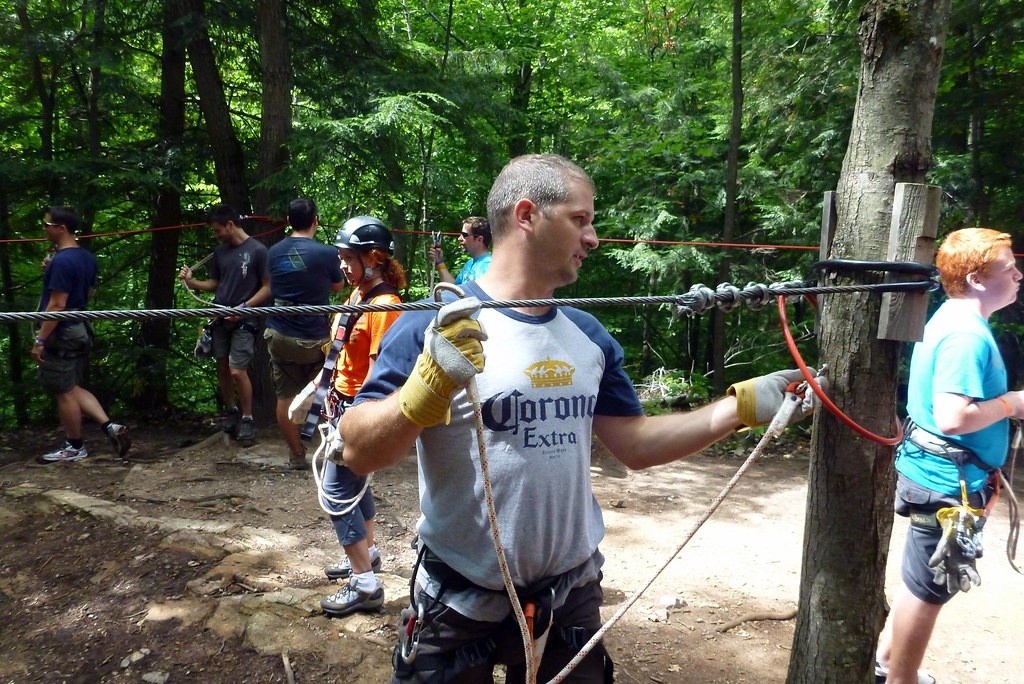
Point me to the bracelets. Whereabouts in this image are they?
[35,338,44,346]
[239,303,243,307]
[436,260,446,270]
[996,396,1011,416]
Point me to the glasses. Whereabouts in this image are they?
[461,232,486,238]
[42,218,59,228]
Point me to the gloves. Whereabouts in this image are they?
[327,415,348,466]
[726,367,830,434]
[928,507,983,594]
[399,296,488,428]
[288,381,318,424]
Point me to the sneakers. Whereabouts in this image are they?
[36,441,88,465]
[324,547,382,578]
[320,570,384,614]
[104,423,131,458]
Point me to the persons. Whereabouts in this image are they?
[338,153,828,684]
[263,198,344,470]
[428,216,492,285]
[876,228,1024,684]
[31,206,132,463]
[288,216,407,613]
[177,205,272,440]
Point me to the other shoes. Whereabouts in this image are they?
[221,406,241,432]
[287,447,306,469]
[238,417,256,439]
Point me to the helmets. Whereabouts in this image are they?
[334,216,394,257]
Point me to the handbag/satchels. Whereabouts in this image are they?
[45,308,89,350]
[194,323,213,360]
